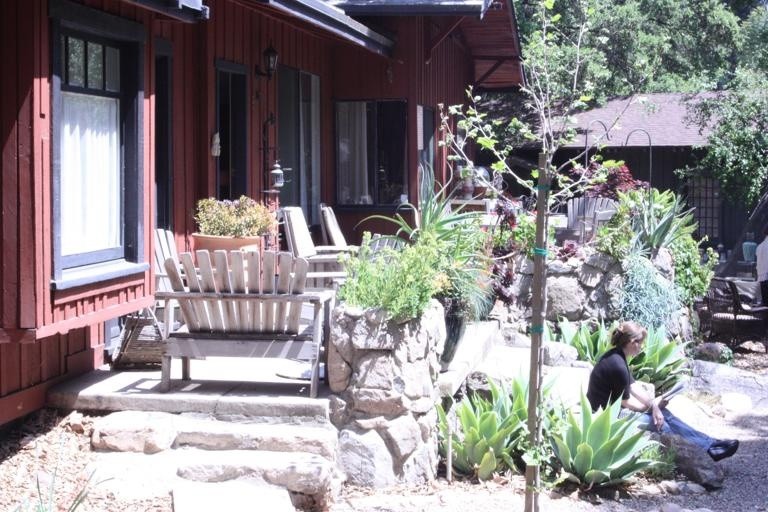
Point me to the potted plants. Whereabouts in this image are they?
[350,165,506,377]
[192,196,275,266]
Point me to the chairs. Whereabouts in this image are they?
[691,258,766,355]
[155,206,364,397]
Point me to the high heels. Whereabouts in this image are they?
[709,441,739,462]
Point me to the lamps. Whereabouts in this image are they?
[256,37,279,79]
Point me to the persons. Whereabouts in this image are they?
[590,321,740,461]
[742,232,757,261]
[754,224,768,354]
